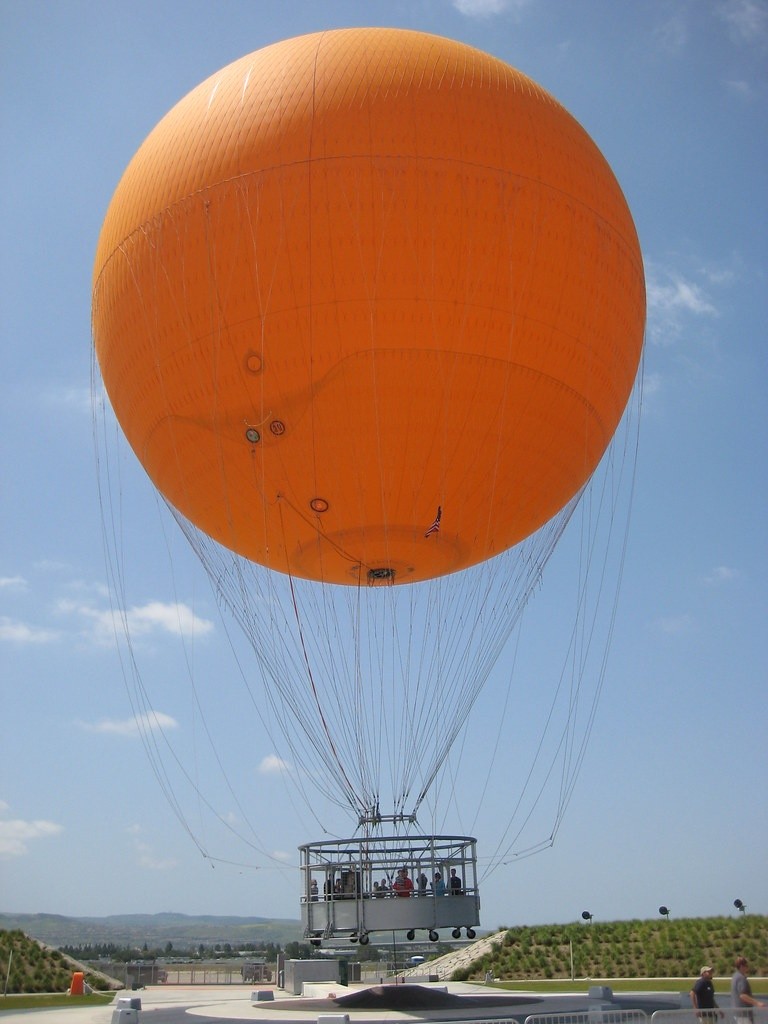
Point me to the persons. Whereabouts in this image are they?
[309,867,461,896]
[690,965,724,1024]
[732,957,767,1024]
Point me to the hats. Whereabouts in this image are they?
[311,880,317,885]
[700,965,714,975]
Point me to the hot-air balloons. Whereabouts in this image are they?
[90,26,647,946]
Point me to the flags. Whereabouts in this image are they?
[424,509,442,538]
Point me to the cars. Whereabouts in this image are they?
[144,965,168,982]
[240,962,272,981]
